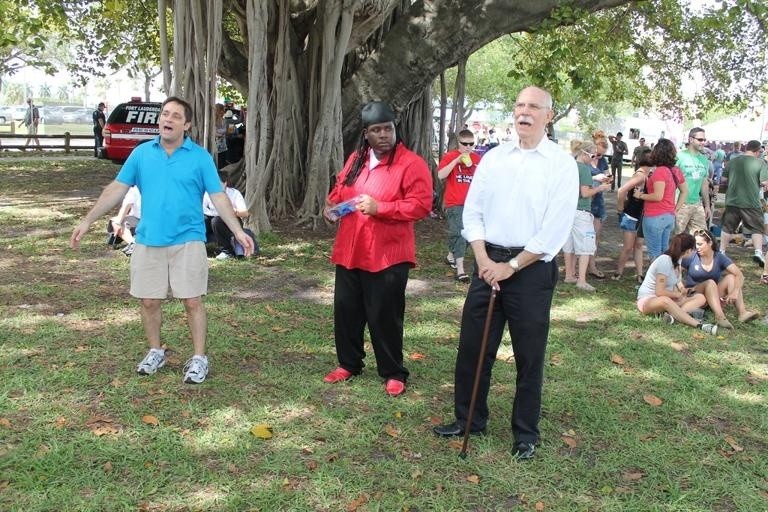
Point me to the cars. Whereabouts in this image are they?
[0,105,94,128]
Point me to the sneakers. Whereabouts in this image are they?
[564,278,577,283]
[738,311,758,321]
[589,269,606,279]
[126,244,134,255]
[386,378,404,396]
[324,368,351,383]
[136,349,164,375]
[456,273,470,284]
[760,274,768,284]
[445,256,456,268]
[664,311,674,324]
[714,316,732,328]
[122,245,129,252]
[182,355,208,384]
[577,282,595,291]
[216,251,231,260]
[701,323,718,335]
[753,251,765,267]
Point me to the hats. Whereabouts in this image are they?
[361,100,395,128]
[98,103,106,108]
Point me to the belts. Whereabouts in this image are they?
[486,245,522,255]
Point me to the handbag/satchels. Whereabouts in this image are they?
[637,219,644,237]
[229,229,258,256]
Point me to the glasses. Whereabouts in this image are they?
[692,136,706,141]
[459,141,474,146]
[694,229,712,240]
[582,148,597,159]
[513,103,549,111]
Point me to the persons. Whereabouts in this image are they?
[322,101,434,396]
[93,103,107,157]
[18,98,42,152]
[564,128,768,332]
[108,184,143,255]
[432,86,580,462]
[71,97,256,384]
[439,121,511,281]
[215,103,246,171]
[203,171,249,262]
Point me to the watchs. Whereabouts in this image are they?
[508,257,519,273]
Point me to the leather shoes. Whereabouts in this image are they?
[433,423,486,437]
[512,441,535,458]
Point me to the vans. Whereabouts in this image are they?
[95,97,246,165]
